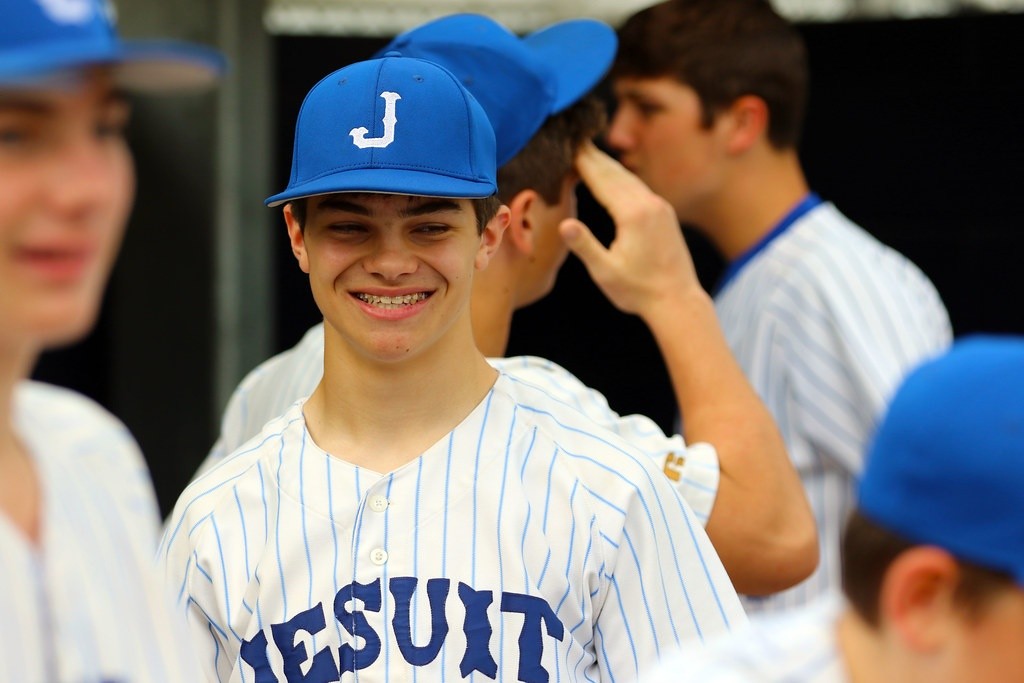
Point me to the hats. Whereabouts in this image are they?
[379,14,617,173]
[263,51,498,206]
[0,0,225,95]
[855,334,1024,586]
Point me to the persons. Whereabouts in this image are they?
[157,0,1024,683]
[0,1,165,683]
